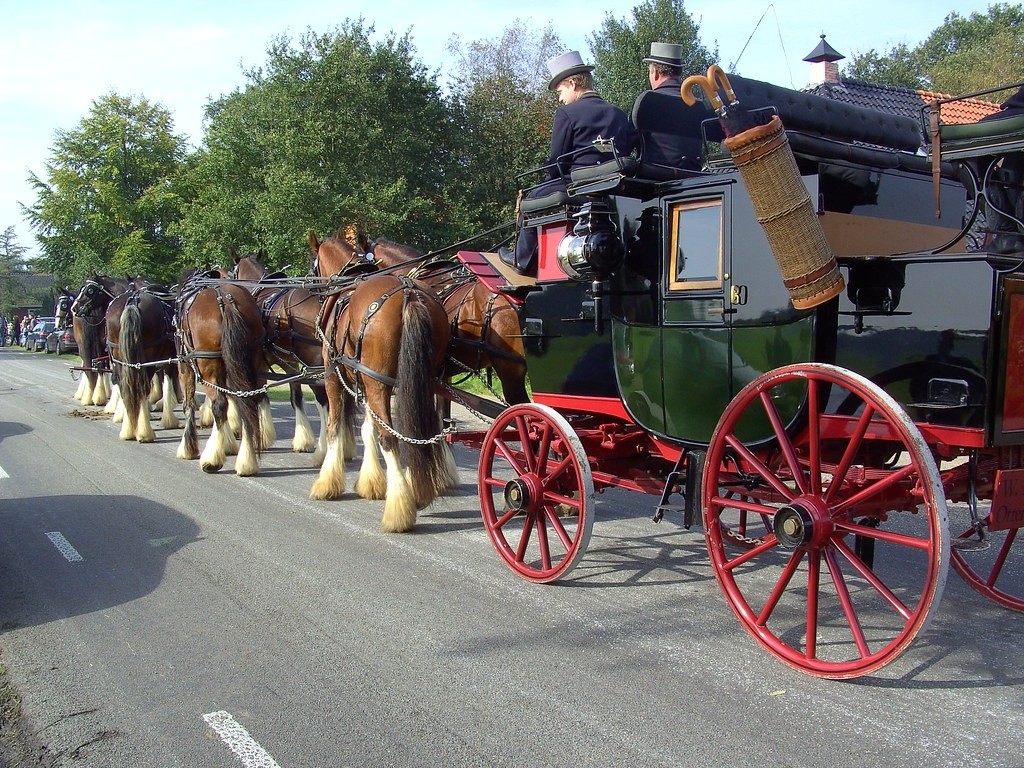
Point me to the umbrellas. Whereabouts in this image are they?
[679,65,772,140]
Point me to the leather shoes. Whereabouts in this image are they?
[499,246,531,276]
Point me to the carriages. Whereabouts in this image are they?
[56,66,1024,678]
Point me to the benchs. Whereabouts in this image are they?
[514,90,712,229]
[919,80,1024,163]
[698,69,967,188]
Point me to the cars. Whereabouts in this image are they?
[7,317,78,356]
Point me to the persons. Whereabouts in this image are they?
[952,85,1024,258]
[630,43,724,171]
[0,313,8,347]
[8,314,41,346]
[498,51,631,274]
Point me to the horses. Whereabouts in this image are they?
[175,262,270,477]
[360,235,581,519]
[227,254,358,468]
[56,271,202,443]
[308,229,450,533]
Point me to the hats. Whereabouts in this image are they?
[546,51,595,90]
[641,42,688,68]
